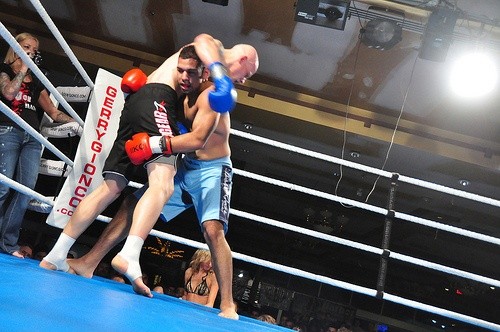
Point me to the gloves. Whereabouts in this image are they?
[208,61,238,113]
[121,68,147,94]
[177,121,188,135]
[125,132,174,166]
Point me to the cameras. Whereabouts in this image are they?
[26,51,43,66]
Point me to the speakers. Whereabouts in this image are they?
[419,8,458,62]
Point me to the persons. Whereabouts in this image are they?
[0,32,75,259]
[67,45,239,321]
[38,33,258,298]
[15,241,388,332]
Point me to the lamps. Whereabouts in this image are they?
[293,0,351,31]
[418,7,459,63]
[358,6,405,52]
